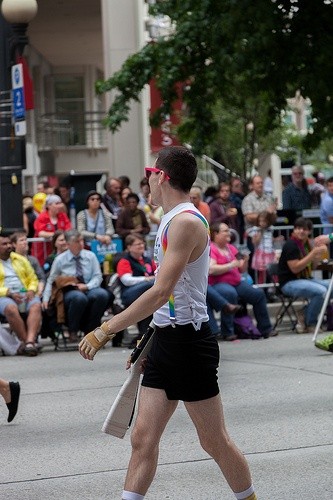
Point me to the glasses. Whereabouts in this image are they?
[144,167,170,180]
[190,196,196,199]
[131,242,145,246]
[89,197,101,201]
[293,172,303,176]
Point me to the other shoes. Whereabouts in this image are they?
[23,342,42,356]
[69,332,78,343]
[226,304,241,314]
[264,331,278,339]
[306,327,323,333]
[214,332,223,339]
[223,335,238,340]
[5,381,20,422]
[295,310,305,333]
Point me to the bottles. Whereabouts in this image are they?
[19,288,28,312]
[103,253,115,274]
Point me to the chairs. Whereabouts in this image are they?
[266,265,304,336]
[47,286,248,352]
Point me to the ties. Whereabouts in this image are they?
[72,256,85,284]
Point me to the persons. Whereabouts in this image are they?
[24,175,166,348]
[77,149,258,500]
[190,166,333,342]
[0,233,47,358]
[0,378,20,422]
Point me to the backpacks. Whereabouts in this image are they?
[235,317,261,339]
